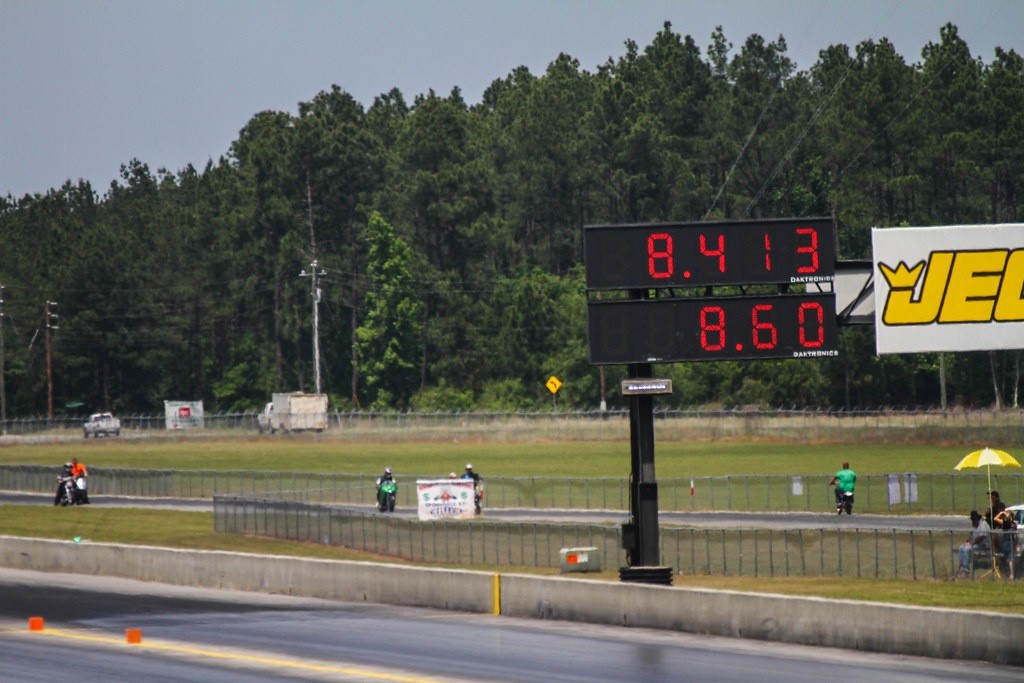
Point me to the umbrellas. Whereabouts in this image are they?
[954,447,1021,580]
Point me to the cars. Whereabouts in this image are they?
[1005,504,1024,556]
[83,413,121,439]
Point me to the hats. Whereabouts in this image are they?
[986,491,999,497]
[970,510,982,519]
[72,458,77,463]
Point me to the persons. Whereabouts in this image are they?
[830,462,857,506]
[955,491,1018,579]
[449,464,483,488]
[375,466,397,504]
[55,458,89,506]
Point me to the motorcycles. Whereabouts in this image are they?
[57,476,78,506]
[832,482,854,515]
[474,480,484,514]
[376,481,398,513]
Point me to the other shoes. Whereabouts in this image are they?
[837,503,842,508]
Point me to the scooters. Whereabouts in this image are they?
[74,475,87,506]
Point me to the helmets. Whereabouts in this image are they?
[384,467,392,479]
[465,464,472,470]
[63,463,74,472]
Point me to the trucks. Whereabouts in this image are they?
[257,392,329,435]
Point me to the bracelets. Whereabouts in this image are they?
[999,511,1003,515]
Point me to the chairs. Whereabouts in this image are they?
[954,529,1024,578]
